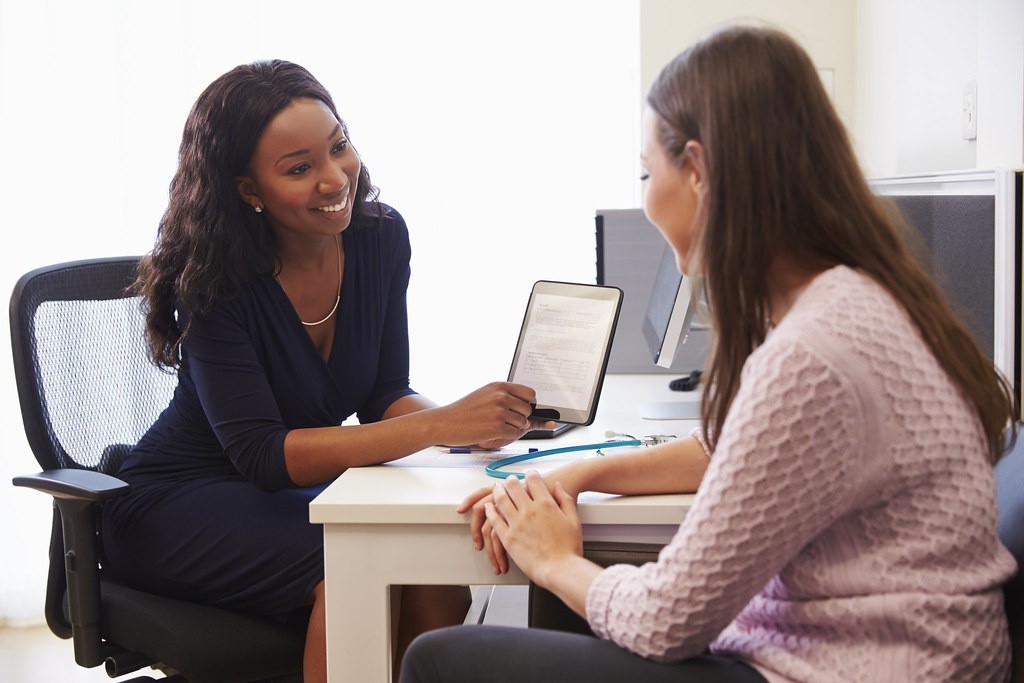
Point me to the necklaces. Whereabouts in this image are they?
[264,234,345,327]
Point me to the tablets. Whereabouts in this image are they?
[506,280,624,426]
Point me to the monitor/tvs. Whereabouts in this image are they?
[640,275,711,419]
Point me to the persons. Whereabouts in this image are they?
[396,22,1024,683]
[99,58,557,683]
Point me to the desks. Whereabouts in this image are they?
[309,373,717,683]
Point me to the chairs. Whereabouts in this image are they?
[9,256,306,683]
